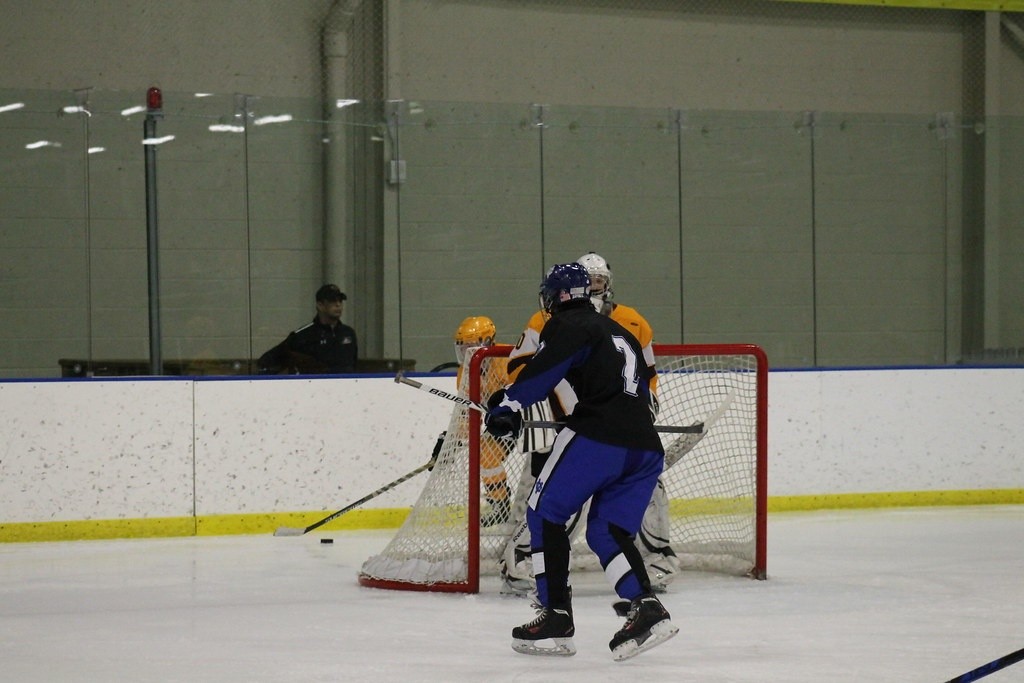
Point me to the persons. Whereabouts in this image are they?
[485,251,681,662]
[428,314,527,528]
[258,283,357,374]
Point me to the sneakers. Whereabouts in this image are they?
[609,592,680,662]
[511,586,575,657]
[480,487,511,532]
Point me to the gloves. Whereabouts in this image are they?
[485,409,524,441]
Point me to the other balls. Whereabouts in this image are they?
[321,538,334,544]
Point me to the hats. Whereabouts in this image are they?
[316,285,347,301]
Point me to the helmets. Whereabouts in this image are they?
[573,254,613,315]
[538,262,592,323]
[454,316,496,364]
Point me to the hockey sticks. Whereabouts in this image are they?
[393,368,709,475]
[943,647,1024,683]
[272,442,469,538]
[484,388,736,435]
